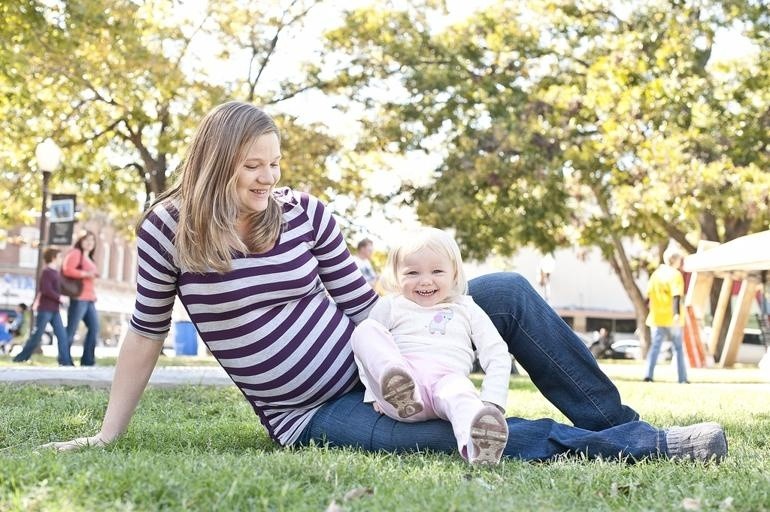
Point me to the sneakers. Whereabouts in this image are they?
[381,366,425,420]
[665,421,728,464]
[466,405,509,468]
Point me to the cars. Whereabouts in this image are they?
[730,327,762,362]
[608,339,640,355]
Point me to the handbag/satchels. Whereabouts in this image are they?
[58,247,83,297]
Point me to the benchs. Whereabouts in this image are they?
[0,310,33,356]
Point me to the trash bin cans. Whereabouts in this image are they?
[174,320,197,356]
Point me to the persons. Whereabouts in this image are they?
[58,230,100,366]
[6,304,28,335]
[643,248,691,384]
[13,249,75,366]
[593,327,617,359]
[32,101,727,464]
[350,227,513,470]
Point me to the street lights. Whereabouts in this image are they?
[22,136,63,356]
